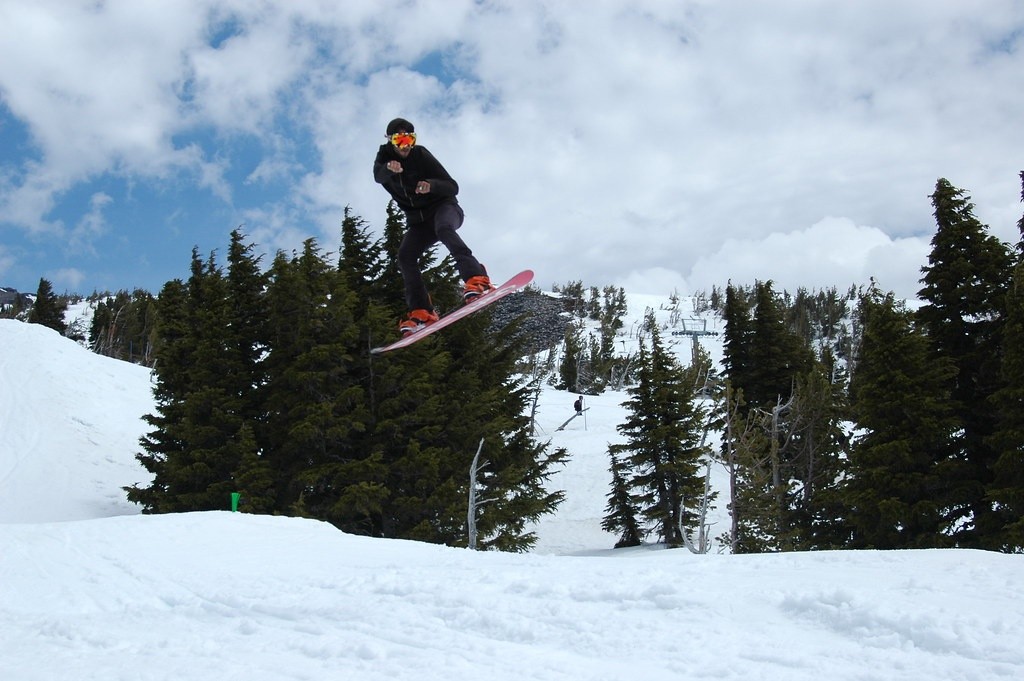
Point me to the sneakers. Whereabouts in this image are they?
[399,309,439,338]
[462,274,496,305]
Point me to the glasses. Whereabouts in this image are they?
[385,132,417,149]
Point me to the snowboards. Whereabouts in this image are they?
[370,269,534,354]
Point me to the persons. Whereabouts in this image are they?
[373,118,496,338]
